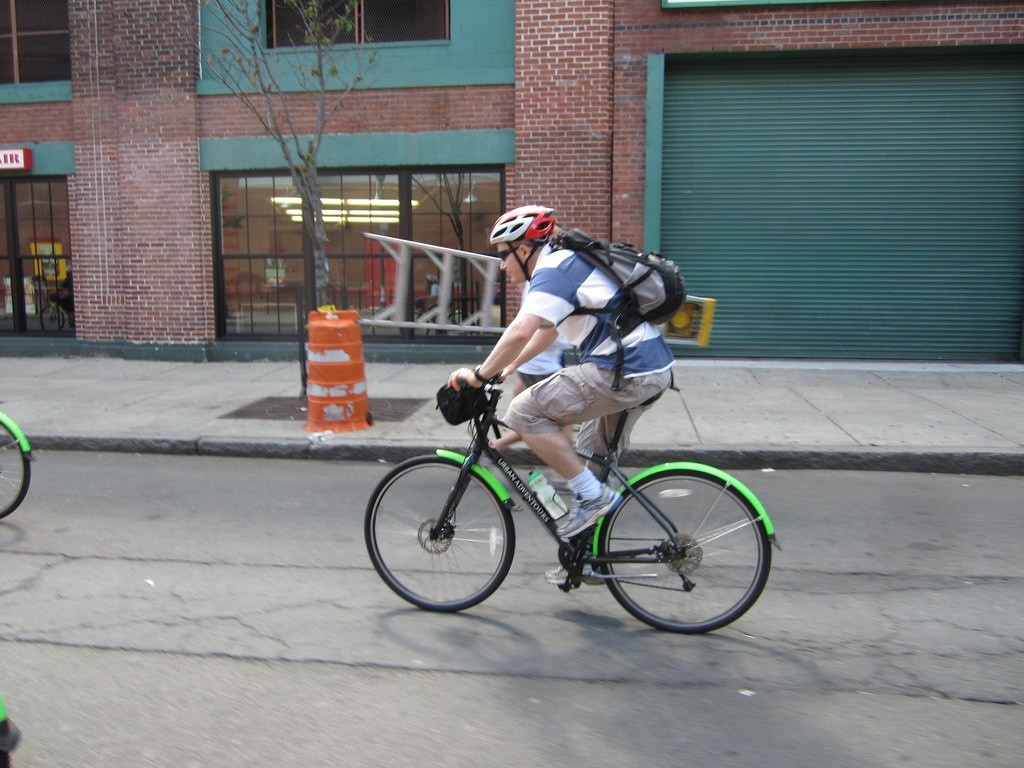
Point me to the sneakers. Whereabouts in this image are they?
[545,555,606,586]
[556,483,624,538]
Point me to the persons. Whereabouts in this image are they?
[425,275,440,322]
[54,264,76,328]
[447,204,677,586]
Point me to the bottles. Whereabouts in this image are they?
[527,471,569,521]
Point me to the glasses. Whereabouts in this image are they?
[497,243,532,260]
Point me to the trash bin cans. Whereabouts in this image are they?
[301,306,373,430]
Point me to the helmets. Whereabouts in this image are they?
[490,201,556,246]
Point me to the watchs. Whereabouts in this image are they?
[474,365,496,383]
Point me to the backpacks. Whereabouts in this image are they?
[547,227,689,328]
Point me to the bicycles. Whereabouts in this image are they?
[361,370,782,635]
[39,295,67,332]
[0,395,37,523]
[0,692,26,768]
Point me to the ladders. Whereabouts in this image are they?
[354,233,715,349]
